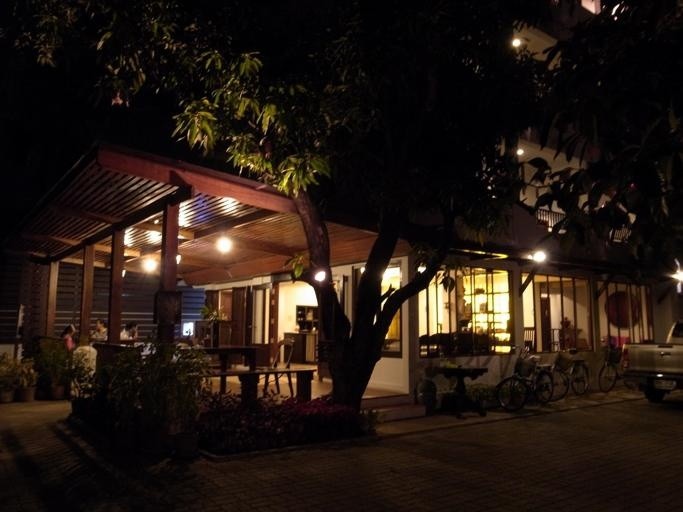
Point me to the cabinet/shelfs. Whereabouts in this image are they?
[295,304,318,330]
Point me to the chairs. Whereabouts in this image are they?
[256,338,295,398]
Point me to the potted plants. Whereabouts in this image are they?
[0,339,73,403]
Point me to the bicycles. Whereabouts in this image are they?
[538,340,590,396]
[494,344,554,410]
[596,335,623,394]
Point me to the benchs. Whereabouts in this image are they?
[198,368,316,402]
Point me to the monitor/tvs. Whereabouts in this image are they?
[179,319,195,336]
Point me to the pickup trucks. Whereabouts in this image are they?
[621,321,683,402]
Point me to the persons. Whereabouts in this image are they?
[91,318,108,342]
[63,324,77,352]
[120,321,139,341]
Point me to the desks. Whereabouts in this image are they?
[185,347,257,396]
[429,367,489,418]
